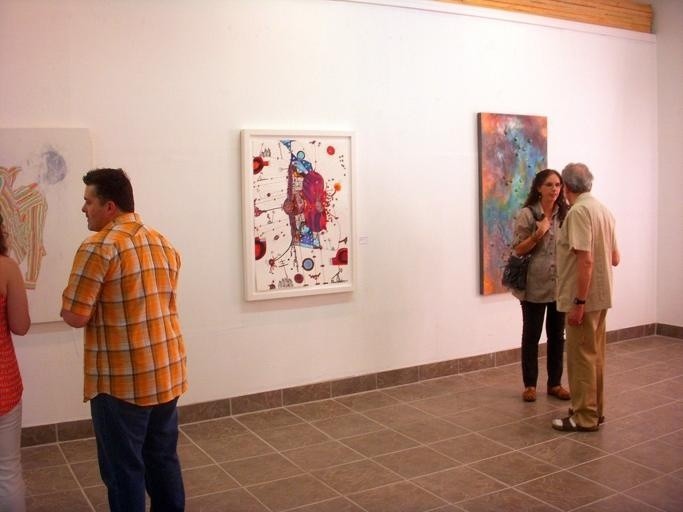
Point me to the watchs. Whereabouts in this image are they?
[572,297,586,305]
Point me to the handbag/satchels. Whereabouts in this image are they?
[502,255,528,289]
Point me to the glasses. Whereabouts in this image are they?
[542,183,562,188]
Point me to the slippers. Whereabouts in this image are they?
[552,407,605,431]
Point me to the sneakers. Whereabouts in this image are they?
[523,386,536,401]
[547,384,570,400]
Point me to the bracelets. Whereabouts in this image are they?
[531,232,541,243]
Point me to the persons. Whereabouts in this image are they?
[509,167,571,402]
[0,214,33,512]
[58,166,190,512]
[551,161,623,433]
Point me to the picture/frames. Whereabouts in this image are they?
[241,128,357,301]
